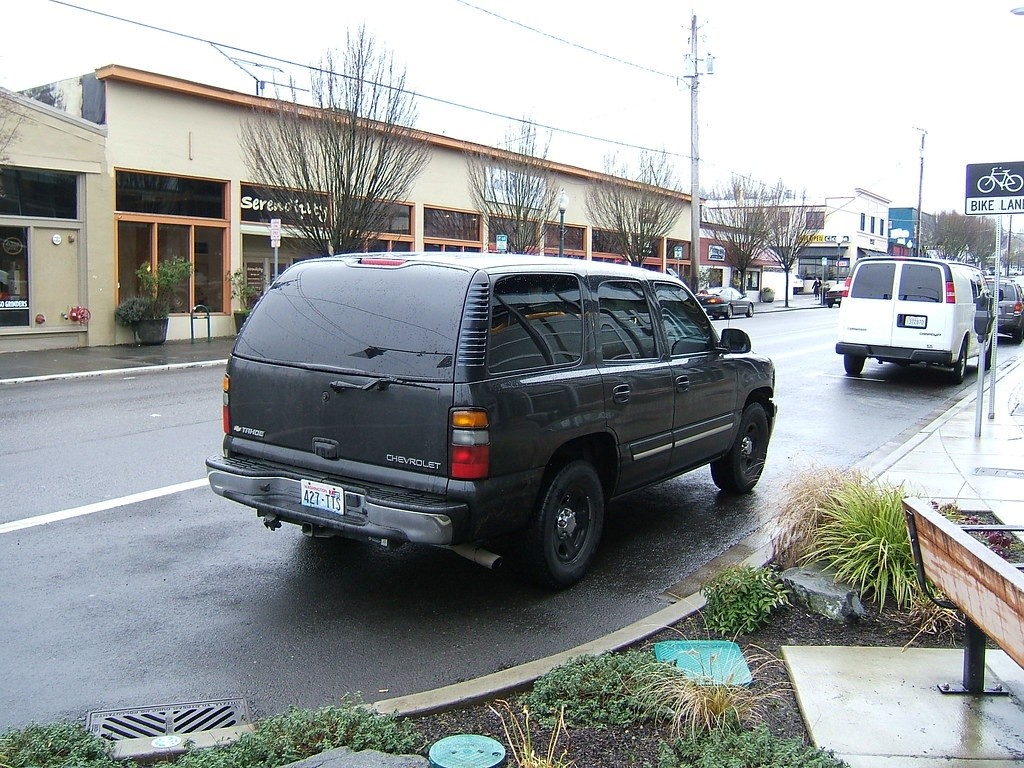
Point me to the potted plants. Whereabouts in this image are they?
[115,255,195,347]
[761,287,775,303]
[224,270,255,336]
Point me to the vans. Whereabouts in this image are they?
[833,252,994,385]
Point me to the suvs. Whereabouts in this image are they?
[203,247,778,594]
[985,276,1024,345]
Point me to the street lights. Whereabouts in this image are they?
[555,190,569,257]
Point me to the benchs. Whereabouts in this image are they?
[901,495,1024,697]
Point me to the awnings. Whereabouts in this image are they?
[857,246,890,256]
[737,247,781,266]
[796,246,846,258]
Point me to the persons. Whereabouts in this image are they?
[811,277,822,300]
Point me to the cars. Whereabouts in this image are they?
[826,282,846,309]
[694,287,755,319]
[980,264,1024,276]
